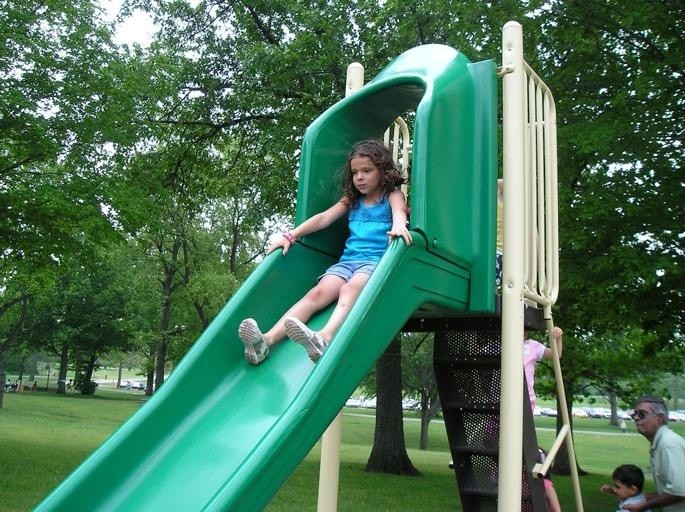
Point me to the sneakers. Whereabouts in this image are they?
[238,318,269,366]
[284,317,328,364]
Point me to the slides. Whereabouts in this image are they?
[35,232,470,512]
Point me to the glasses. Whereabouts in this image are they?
[630,412,659,420]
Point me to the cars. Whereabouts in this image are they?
[533,405,635,420]
[345,395,377,408]
[668,409,685,421]
[120,381,155,393]
[401,398,423,411]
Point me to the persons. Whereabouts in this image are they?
[521,444,564,512]
[621,396,684,511]
[600,462,654,512]
[238,140,412,364]
[467,322,566,486]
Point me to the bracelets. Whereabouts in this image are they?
[280,231,297,245]
